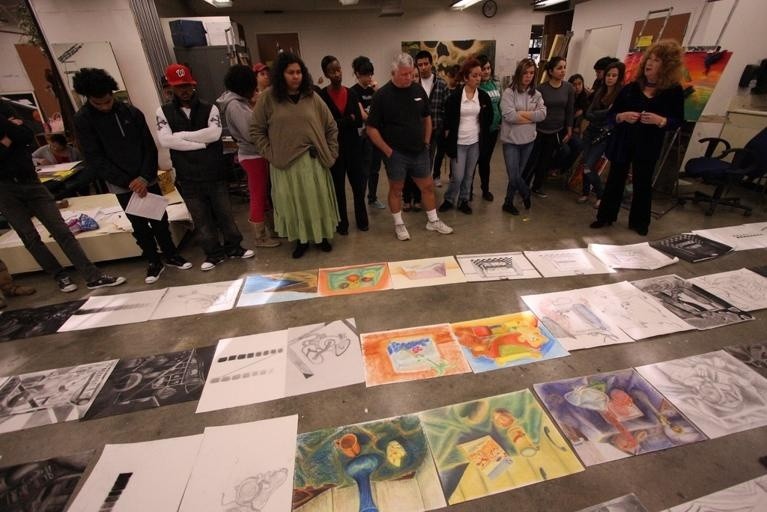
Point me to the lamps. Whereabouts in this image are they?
[452,0,482,10]
[534,0,566,9]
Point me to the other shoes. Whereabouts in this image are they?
[433,178,442,186]
[502,181,565,217]
[629,221,646,236]
[576,196,588,204]
[591,221,613,228]
[593,199,601,208]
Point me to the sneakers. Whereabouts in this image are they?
[394,192,493,240]
[292,197,386,258]
[0,245,256,309]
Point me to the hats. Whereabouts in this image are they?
[165,64,197,86]
[252,63,269,72]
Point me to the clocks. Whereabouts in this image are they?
[483,0,497,18]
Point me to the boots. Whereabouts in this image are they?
[247,217,281,248]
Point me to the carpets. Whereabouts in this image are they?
[621,190,680,219]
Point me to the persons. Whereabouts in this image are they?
[1,109,127,291]
[72,66,193,284]
[31,133,90,199]
[155,61,255,271]
[0,256,37,308]
[590,38,685,235]
[215,51,626,257]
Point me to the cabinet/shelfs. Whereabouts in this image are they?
[712,109,767,185]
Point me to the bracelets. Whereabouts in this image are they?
[659,116,667,130]
[424,141,432,148]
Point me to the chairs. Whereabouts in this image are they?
[678,127,767,215]
